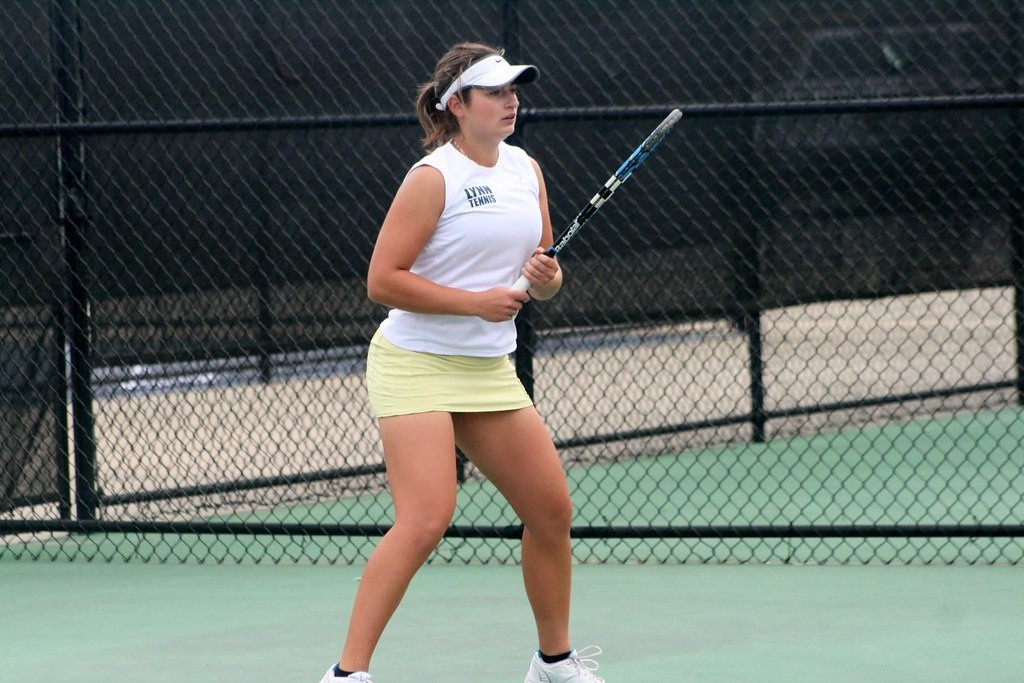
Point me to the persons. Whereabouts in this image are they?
[318,41,608,683]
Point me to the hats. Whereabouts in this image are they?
[435,52,540,112]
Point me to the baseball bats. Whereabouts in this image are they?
[499,108,681,290]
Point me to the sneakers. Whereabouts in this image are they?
[320,661,372,683]
[525,646,604,683]
